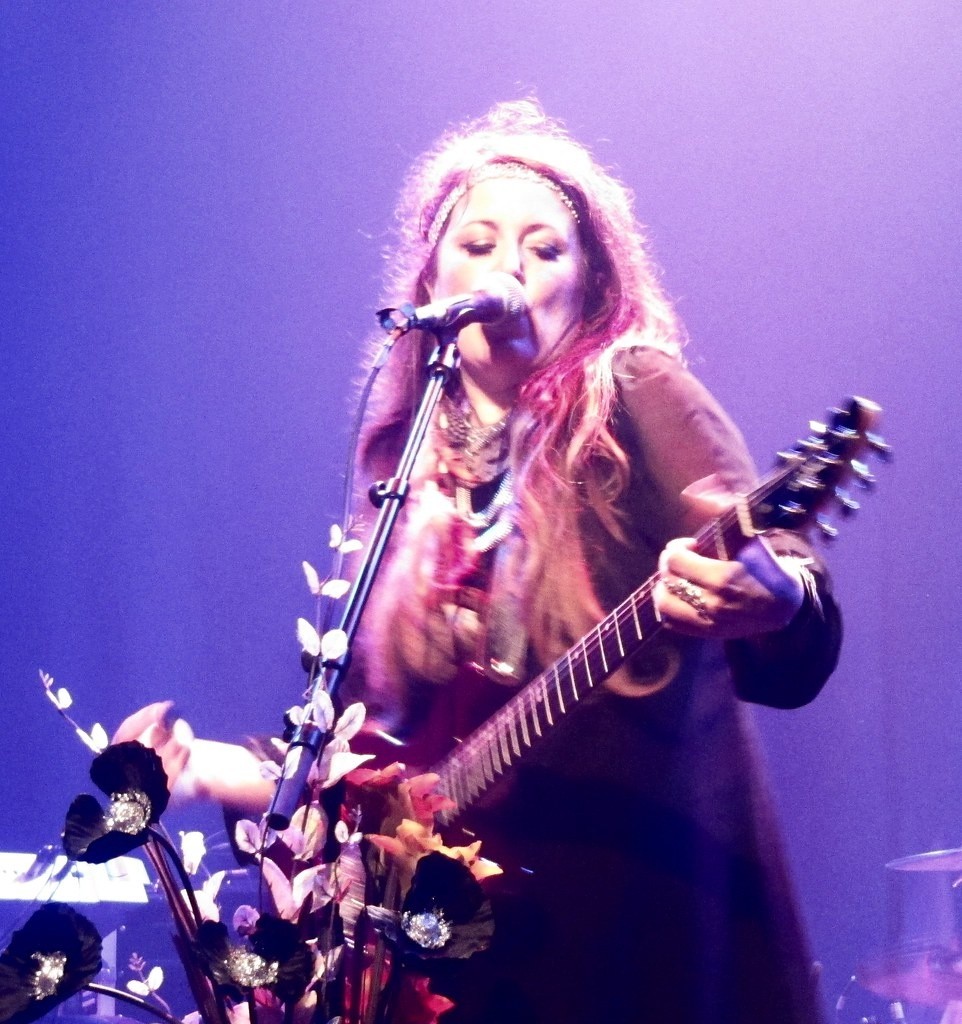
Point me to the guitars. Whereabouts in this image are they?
[249,391,895,1024]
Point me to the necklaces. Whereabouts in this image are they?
[452,406,511,523]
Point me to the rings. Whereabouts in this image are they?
[669,576,707,619]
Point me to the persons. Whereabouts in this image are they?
[108,80,843,1022]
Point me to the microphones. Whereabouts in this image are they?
[395,272,526,336]
